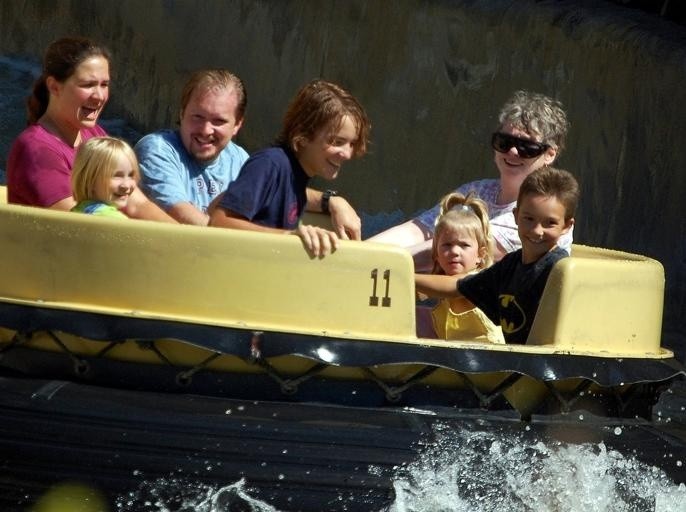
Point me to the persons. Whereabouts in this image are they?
[7,37,182,226]
[414,166,582,343]
[133,68,361,242]
[416,190,506,344]
[69,136,139,218]
[360,89,573,339]
[209,78,370,257]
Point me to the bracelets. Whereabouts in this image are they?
[322,188,340,217]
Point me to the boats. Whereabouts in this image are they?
[0,183,686,512]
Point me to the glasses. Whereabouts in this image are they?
[490,131,548,159]
